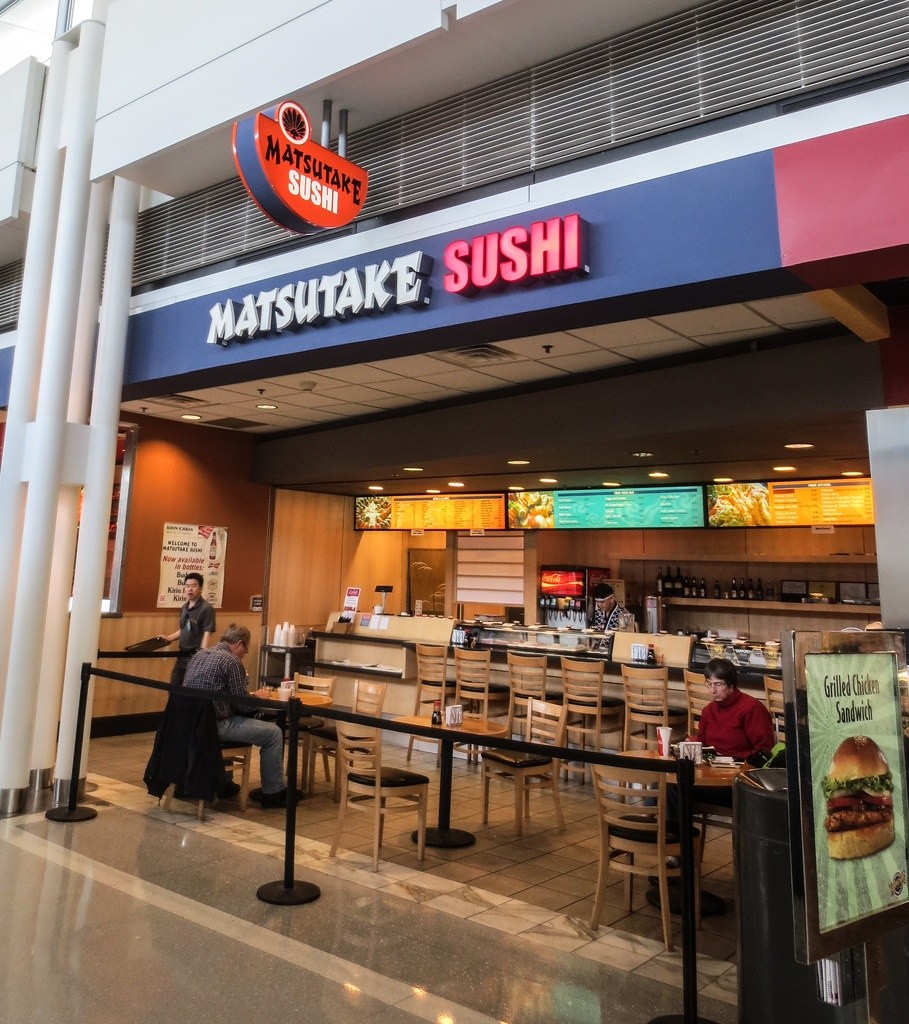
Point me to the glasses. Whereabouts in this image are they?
[704,679,728,690]
[239,640,249,655]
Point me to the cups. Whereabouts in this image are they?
[277,680,295,701]
[274,622,296,653]
[656,726,673,757]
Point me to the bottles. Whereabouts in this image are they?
[656,565,775,601]
[647,644,656,664]
[432,701,442,724]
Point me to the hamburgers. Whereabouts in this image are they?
[820,734,895,860]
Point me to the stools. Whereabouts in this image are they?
[405,644,787,784]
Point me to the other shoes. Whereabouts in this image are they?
[217,781,241,799]
[262,787,306,807]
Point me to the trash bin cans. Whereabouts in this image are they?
[732,767,836,1024]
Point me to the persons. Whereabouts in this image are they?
[649,658,774,883]
[158,573,217,686]
[182,622,287,809]
[592,583,628,649]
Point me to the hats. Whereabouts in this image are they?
[593,583,614,601]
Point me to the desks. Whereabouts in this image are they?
[390,715,507,848]
[248,689,333,804]
[615,750,758,917]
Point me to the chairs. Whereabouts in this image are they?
[144,672,786,951]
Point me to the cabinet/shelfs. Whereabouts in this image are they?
[312,629,418,680]
[259,645,315,685]
